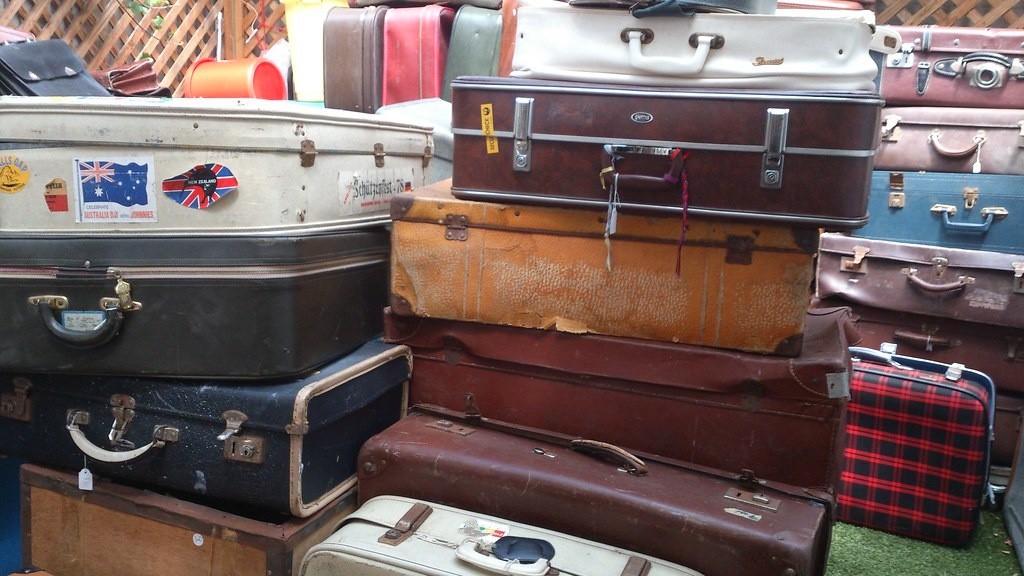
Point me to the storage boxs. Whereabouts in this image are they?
[184,56,287,102]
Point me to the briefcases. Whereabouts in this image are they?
[1,1,1021,573]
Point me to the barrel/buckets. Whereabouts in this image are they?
[183,56,289,104]
